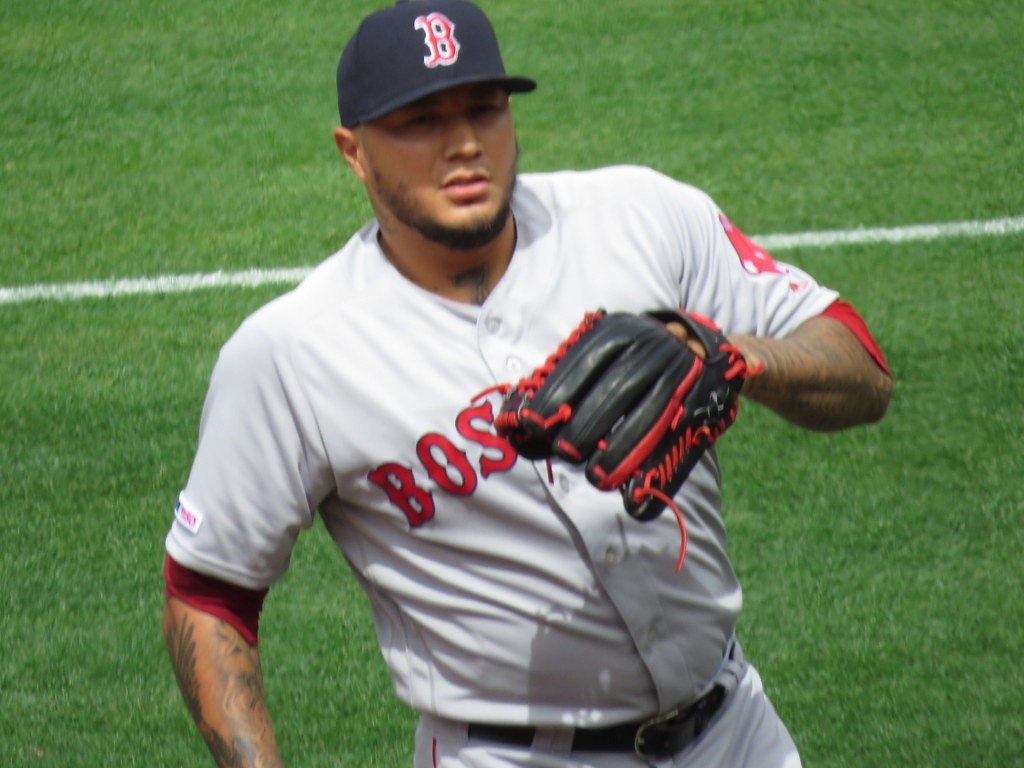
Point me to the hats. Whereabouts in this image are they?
[335,0,537,128]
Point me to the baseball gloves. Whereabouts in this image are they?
[492,307,766,571]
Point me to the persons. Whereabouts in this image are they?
[162,2,894,768]
